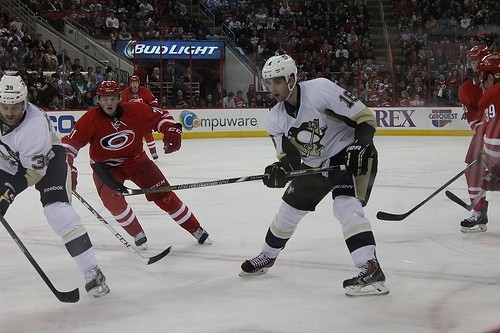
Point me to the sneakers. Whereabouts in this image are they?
[238,253,276,277]
[460,210,488,233]
[134,229,148,249]
[84,265,110,299]
[192,226,212,244]
[343,259,390,296]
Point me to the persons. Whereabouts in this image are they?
[0,74,106,293]
[241,53,386,290]
[61,80,209,245]
[457,45,490,227]
[477,52,500,191]
[119,75,159,160]
[0,0,500,115]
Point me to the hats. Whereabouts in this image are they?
[95,64,102,69]
[154,68,159,70]
[195,90,200,94]
[13,47,18,50]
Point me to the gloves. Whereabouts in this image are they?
[263,162,295,188]
[476,149,495,172]
[343,136,374,176]
[70,166,79,196]
[0,182,17,217]
[163,123,183,154]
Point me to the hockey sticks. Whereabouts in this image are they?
[0,211,80,303]
[71,189,173,265]
[445,188,487,211]
[376,158,478,221]
[91,162,347,196]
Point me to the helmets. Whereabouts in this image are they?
[96,80,122,103]
[476,53,500,82]
[262,53,298,90]
[466,44,492,69]
[0,73,29,126]
[130,75,140,85]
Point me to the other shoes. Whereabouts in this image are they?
[152,153,158,159]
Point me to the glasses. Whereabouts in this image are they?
[25,34,28,35]
